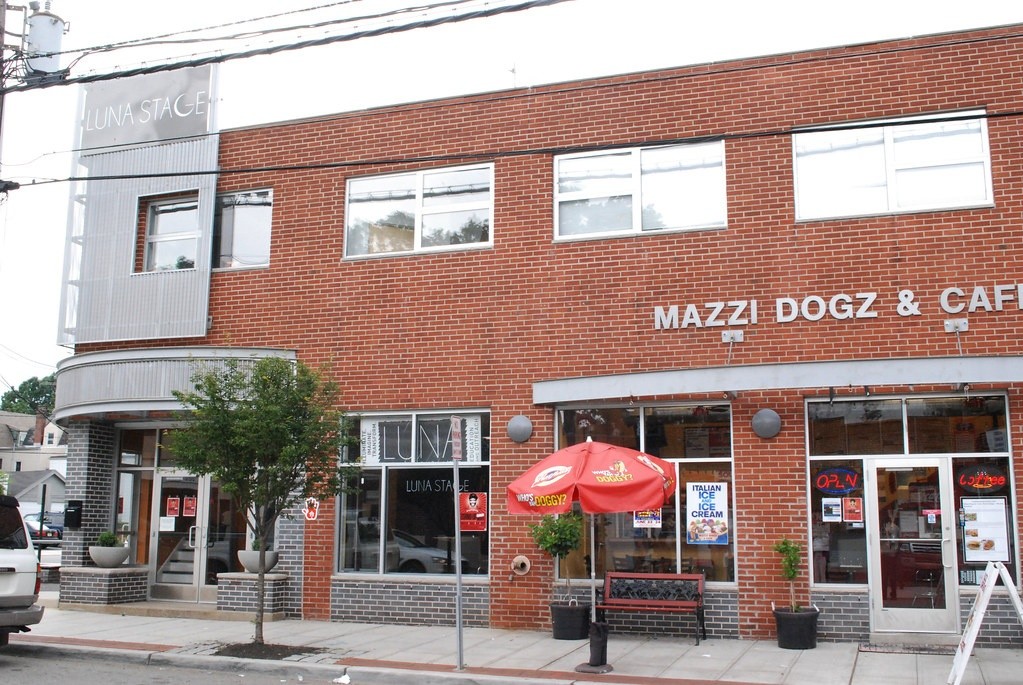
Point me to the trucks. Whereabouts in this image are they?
[897,482,966,569]
[0,495,45,645]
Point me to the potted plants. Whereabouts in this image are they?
[769,536,823,652]
[236,535,280,572]
[87,530,132,568]
[527,511,591,640]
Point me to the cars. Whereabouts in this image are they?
[345,521,400,571]
[392,529,469,573]
[24,511,65,540]
[183,540,231,585]
[23,518,61,549]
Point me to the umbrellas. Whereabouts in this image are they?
[507,437,677,623]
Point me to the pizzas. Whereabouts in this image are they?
[688,519,728,536]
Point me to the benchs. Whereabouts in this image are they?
[594,570,708,649]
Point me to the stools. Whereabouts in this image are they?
[911,563,943,608]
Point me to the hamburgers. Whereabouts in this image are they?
[967,541,981,550]
